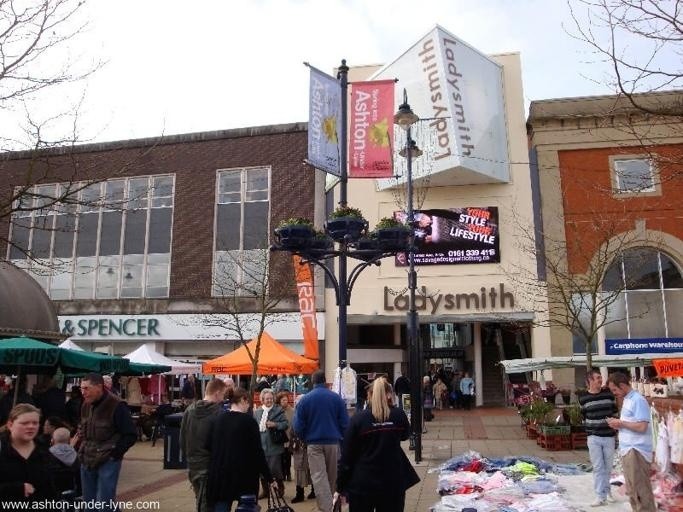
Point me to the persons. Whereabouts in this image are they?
[276,390,294,482]
[459,371,476,410]
[48,425,80,494]
[292,370,349,512]
[0,403,61,512]
[290,392,317,504]
[431,378,447,409]
[333,377,422,512]
[421,375,433,422]
[605,371,655,511]
[577,365,620,506]
[204,386,278,509]
[253,388,290,499]
[177,377,228,510]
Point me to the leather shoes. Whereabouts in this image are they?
[278,487,287,498]
[259,491,271,499]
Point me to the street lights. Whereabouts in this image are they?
[397,142,423,450]
[392,89,424,462]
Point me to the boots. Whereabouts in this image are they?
[291,485,305,504]
[307,484,317,500]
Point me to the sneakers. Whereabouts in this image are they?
[607,493,616,505]
[590,497,608,506]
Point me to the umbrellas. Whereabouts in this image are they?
[129,360,172,376]
[56,336,86,352]
[2,334,133,378]
[75,372,137,512]
[200,330,319,377]
[121,343,204,379]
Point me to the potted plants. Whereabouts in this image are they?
[369,213,411,250]
[323,205,368,243]
[303,228,334,250]
[356,229,380,252]
[271,213,318,247]
